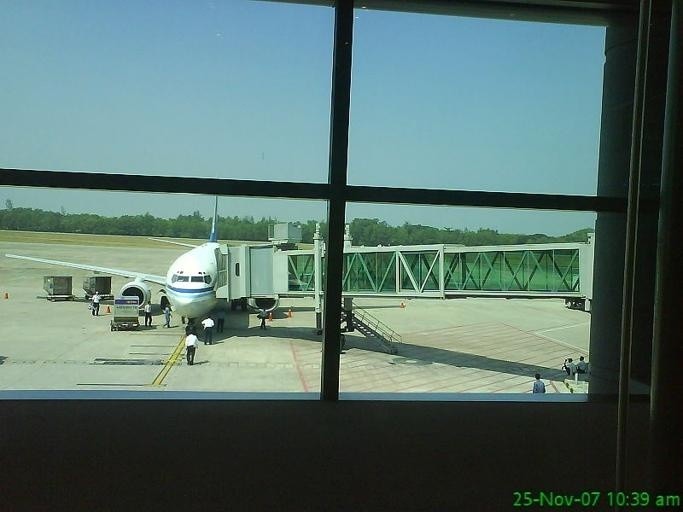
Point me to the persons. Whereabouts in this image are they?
[533,373,545,394]
[90,291,101,316]
[185,330,198,364]
[143,301,153,325]
[164,304,172,329]
[258,308,267,331]
[201,316,215,345]
[576,357,586,373]
[216,308,225,331]
[561,357,577,375]
[185,321,197,336]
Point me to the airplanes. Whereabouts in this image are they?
[6,196,325,326]
